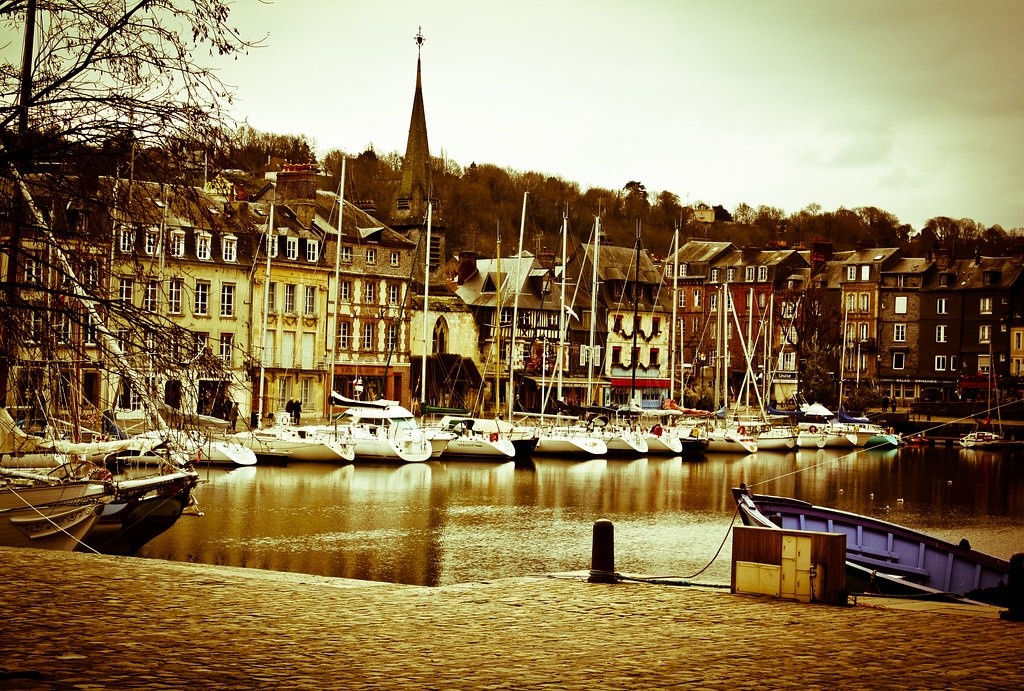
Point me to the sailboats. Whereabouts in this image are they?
[0,143,199,557]
[209,203,357,463]
[374,201,517,461]
[275,155,433,463]
[958,341,1005,447]
[470,191,899,457]
[53,188,261,468]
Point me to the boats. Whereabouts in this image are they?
[732,482,1011,608]
[1,459,119,549]
[334,378,458,459]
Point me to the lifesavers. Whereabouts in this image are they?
[809,425,818,434]
[86,467,113,484]
[655,426,662,436]
[490,433,498,442]
[737,426,745,435]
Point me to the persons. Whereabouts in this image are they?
[223,397,239,430]
[284,396,302,425]
